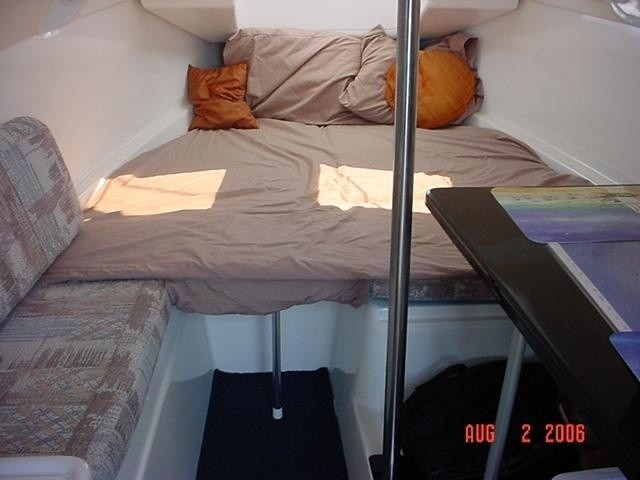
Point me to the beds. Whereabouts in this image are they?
[61,114,599,420]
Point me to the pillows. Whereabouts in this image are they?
[187,62,260,131]
[384,48,477,128]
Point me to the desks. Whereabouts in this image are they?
[424,183,637,480]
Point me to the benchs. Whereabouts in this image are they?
[1,113,214,478]
